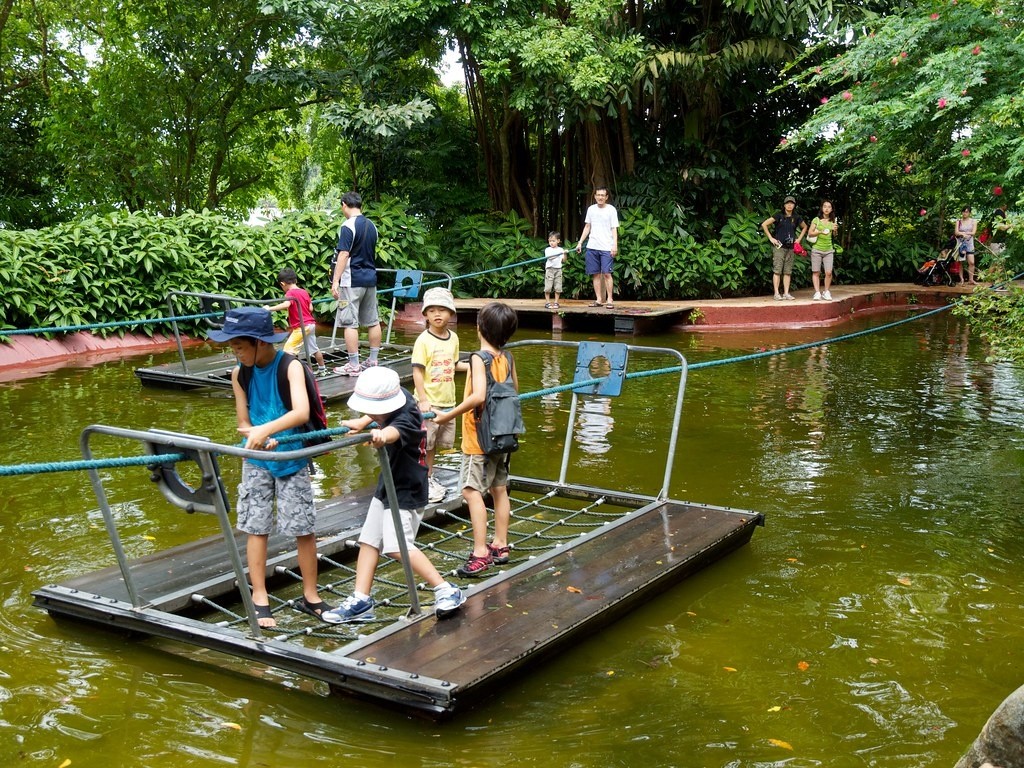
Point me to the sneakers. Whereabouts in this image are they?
[321,595,378,624]
[363,358,379,369]
[333,362,362,377]
[435,583,468,618]
[427,477,448,504]
[313,367,329,376]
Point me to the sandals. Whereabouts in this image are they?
[486,543,510,563]
[253,601,277,630]
[457,550,496,574]
[298,595,332,622]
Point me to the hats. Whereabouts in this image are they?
[347,366,407,415]
[794,242,807,256]
[422,288,456,317]
[208,306,290,345]
[784,196,796,203]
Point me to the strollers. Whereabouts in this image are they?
[912,235,967,287]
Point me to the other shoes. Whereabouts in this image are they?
[783,294,796,301]
[773,294,783,301]
[988,285,1009,292]
[813,290,832,302]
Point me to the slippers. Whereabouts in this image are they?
[606,302,614,309]
[545,302,561,309]
[588,301,604,308]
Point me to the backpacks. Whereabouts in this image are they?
[979,226,995,242]
[469,351,526,457]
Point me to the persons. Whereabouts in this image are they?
[207,306,335,628]
[762,196,807,301]
[954,205,980,287]
[328,191,382,377]
[431,302,519,578]
[987,203,1014,292]
[576,187,620,309]
[261,268,328,377]
[544,231,569,309]
[808,200,838,301]
[321,366,467,624]
[917,249,949,273]
[410,287,469,504]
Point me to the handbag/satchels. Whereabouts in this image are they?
[806,217,819,243]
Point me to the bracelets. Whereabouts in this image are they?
[578,241,583,244]
